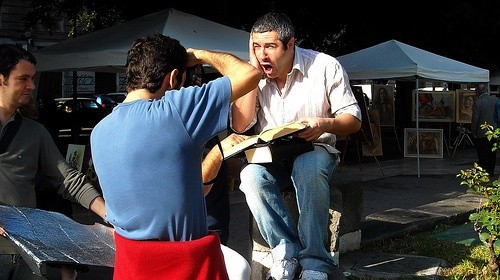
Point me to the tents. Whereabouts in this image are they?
[335,40,490,178]
[34,7,250,73]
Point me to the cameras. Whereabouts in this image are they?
[183,64,206,88]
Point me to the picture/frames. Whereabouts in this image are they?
[404,129,443,158]
[412,91,455,122]
[456,90,476,123]
[371,85,395,126]
[362,110,383,155]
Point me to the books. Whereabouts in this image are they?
[220,121,315,164]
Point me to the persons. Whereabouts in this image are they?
[90,32,262,280]
[0,43,106,280]
[471,83,500,175]
[228,12,361,280]
[201,133,252,280]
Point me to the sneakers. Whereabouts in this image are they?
[298,269,329,280]
[265,256,300,280]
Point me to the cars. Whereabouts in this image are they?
[53,91,128,129]
